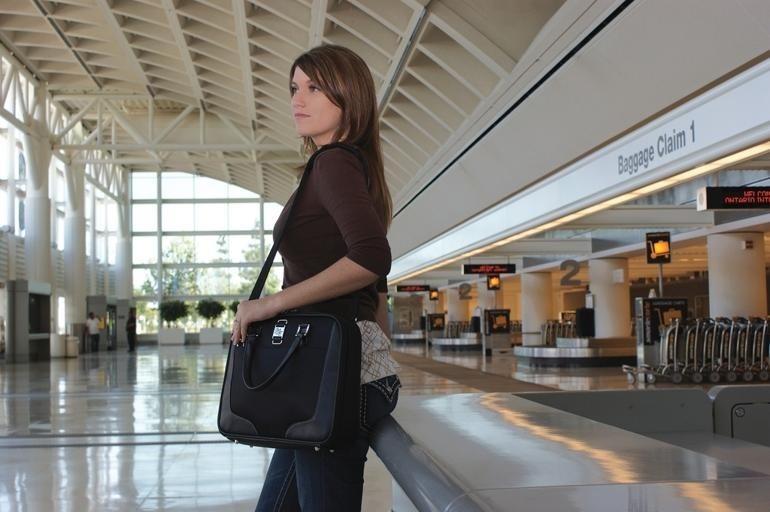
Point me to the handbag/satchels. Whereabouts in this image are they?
[218,313,361,454]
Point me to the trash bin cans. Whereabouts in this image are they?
[66,337,80,357]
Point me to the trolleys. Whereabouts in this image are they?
[622,316,770,384]
[530,320,577,369]
[439,320,471,352]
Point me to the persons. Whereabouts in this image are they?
[126,310,137,351]
[86,312,102,352]
[218,46,403,512]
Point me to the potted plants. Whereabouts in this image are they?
[157,297,226,344]
